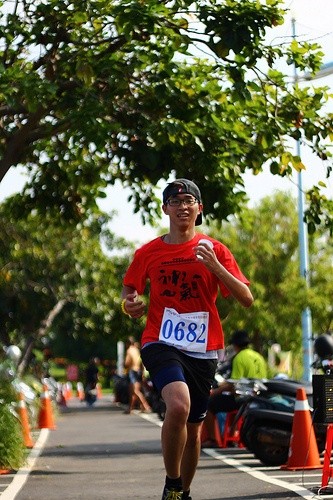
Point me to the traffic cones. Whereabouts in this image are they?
[34,385,58,430]
[13,391,35,450]
[58,381,104,408]
[279,388,326,470]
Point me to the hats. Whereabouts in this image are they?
[162,178,201,205]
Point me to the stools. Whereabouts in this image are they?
[222,410,243,449]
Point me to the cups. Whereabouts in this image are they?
[196,239,214,259]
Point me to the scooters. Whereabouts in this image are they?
[225,334,333,466]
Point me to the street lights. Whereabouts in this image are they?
[291,17,332,408]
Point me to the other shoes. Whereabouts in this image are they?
[161,486,183,500]
[181,488,192,500]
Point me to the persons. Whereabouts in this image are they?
[202,330,266,449]
[120,179,254,500]
[114,368,166,414]
[123,335,153,414]
[81,357,101,407]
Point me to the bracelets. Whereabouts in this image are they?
[210,389,215,397]
[121,299,130,315]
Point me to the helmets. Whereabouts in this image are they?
[315,335,333,361]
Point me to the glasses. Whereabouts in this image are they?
[166,197,199,208]
